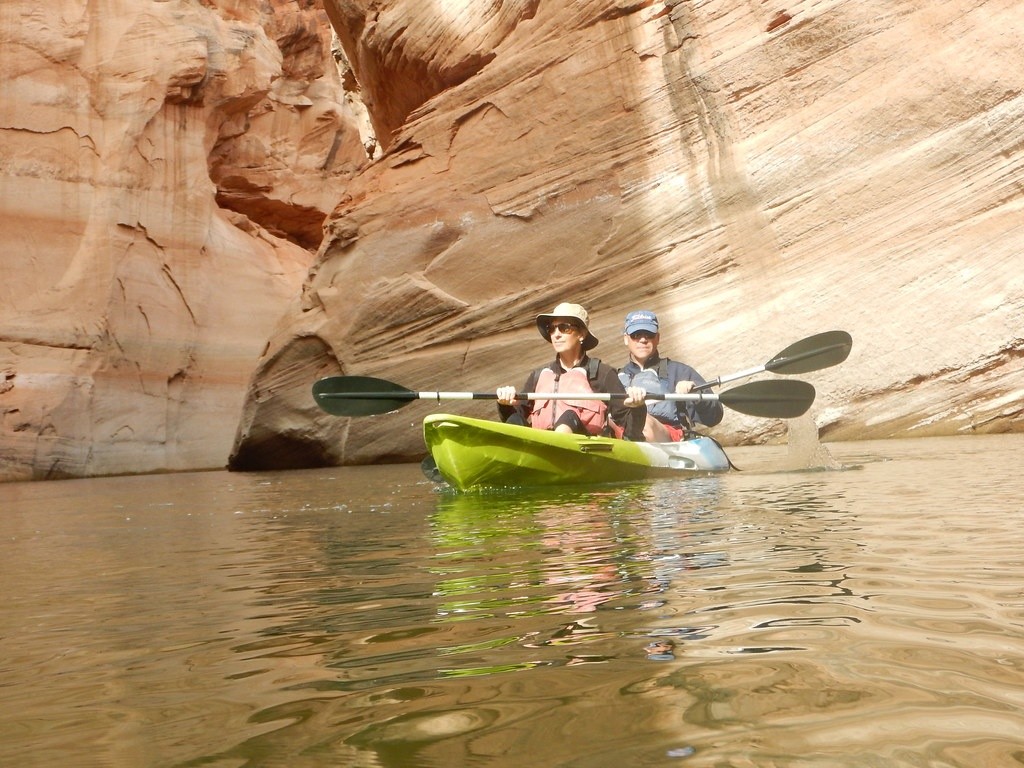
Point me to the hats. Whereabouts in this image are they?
[624,311,658,335]
[536,302,599,351]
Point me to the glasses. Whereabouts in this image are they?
[544,323,581,334]
[628,330,657,340]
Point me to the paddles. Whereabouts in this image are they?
[639,331,853,407]
[311,375,816,420]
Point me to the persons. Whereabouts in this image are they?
[618,309,724,443]
[497,302,647,443]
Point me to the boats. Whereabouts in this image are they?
[425,412,733,493]
